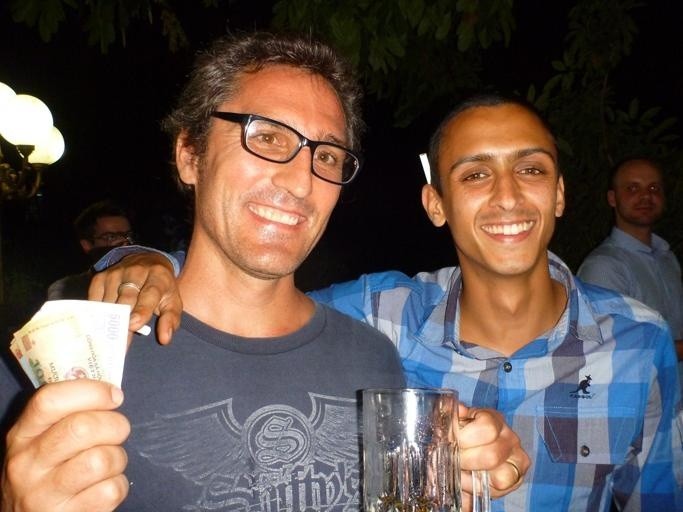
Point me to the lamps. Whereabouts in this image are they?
[0,82,66,209]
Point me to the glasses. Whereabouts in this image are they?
[211,111,360,186]
[91,230,135,241]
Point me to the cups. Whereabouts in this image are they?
[360,386,492,512]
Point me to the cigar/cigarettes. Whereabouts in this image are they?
[418,152,432,185]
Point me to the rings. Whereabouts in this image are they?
[117,281,140,297]
[506,459,520,481]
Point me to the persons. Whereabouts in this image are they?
[45,198,136,309]
[573,155,682,358]
[78,90,683,510]
[0,25,532,512]
